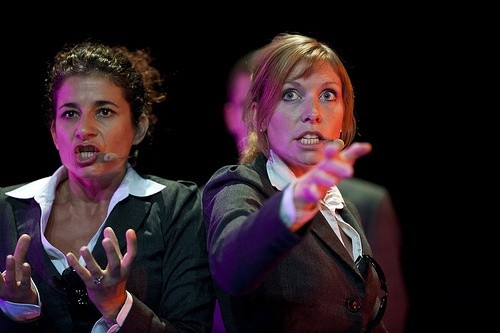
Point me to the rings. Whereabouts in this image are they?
[93,276,103,285]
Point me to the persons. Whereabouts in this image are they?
[0,41,216,333]
[202,32,411,333]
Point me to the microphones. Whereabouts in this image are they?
[104,153,132,162]
[333,139,344,149]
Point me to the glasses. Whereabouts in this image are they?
[355,255,388,333]
[66,279,96,333]
